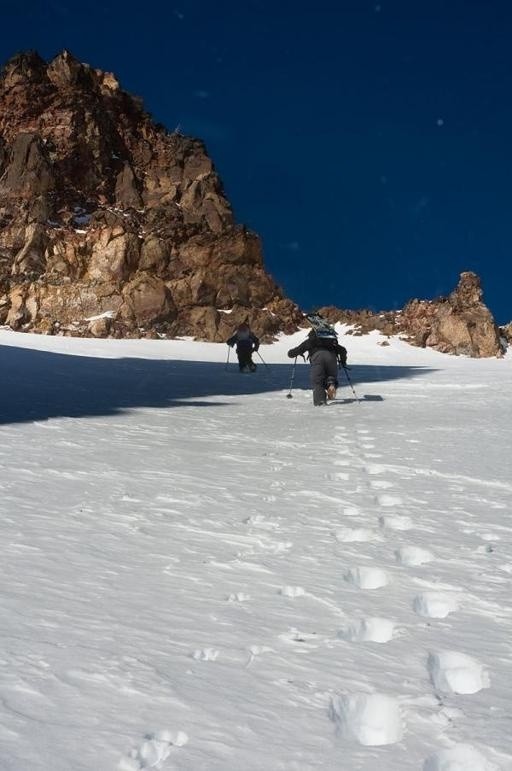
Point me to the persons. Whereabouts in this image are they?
[226,324,260,372]
[287,328,348,406]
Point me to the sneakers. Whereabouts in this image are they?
[316,401,327,407]
[325,376,338,400]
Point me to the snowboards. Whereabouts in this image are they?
[306,313,336,341]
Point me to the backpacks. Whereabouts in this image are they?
[308,326,338,350]
